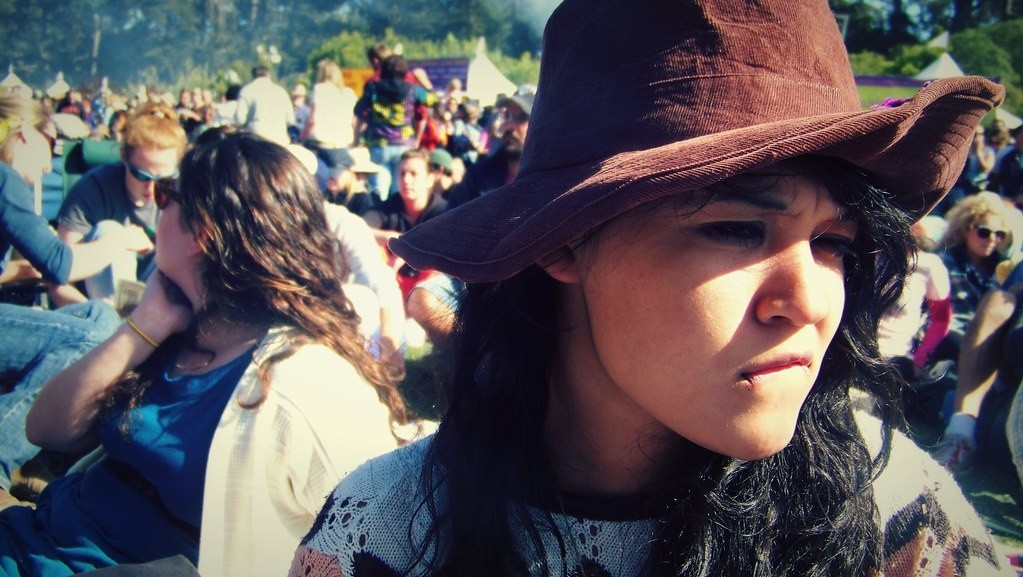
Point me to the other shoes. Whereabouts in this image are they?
[930,430,978,469]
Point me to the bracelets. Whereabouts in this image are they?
[126,317,160,348]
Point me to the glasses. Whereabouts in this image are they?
[973,225,1005,240]
[126,152,181,183]
[36,127,56,154]
[153,177,191,211]
[503,110,534,123]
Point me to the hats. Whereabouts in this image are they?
[429,148,454,171]
[388,0,1006,282]
[316,146,355,178]
[508,85,538,116]
[349,146,379,174]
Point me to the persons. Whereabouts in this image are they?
[287,0,1020,577]
[0,43,1023,515]
[0,134,408,577]
